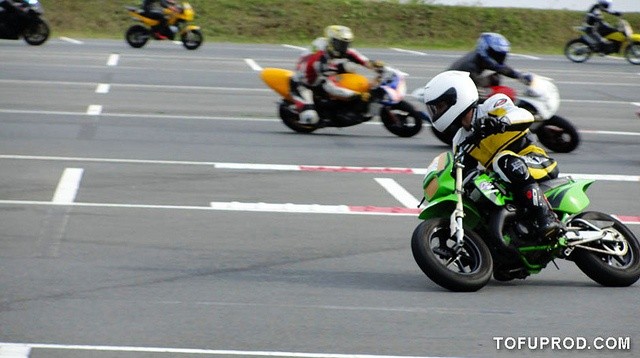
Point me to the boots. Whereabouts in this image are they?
[522,183,561,238]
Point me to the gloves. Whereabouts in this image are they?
[473,115,505,138]
[523,74,534,86]
[615,11,621,16]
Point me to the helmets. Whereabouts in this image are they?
[596,1,613,9]
[424,70,479,144]
[324,25,354,58]
[478,32,510,65]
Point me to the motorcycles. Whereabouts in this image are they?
[257,67,423,139]
[0,0,50,47]
[564,19,640,65]
[125,2,204,50]
[410,123,640,294]
[413,75,580,154]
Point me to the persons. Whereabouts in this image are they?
[144,0,178,35]
[424,70,566,239]
[290,24,383,124]
[448,31,532,87]
[586,0,621,43]
